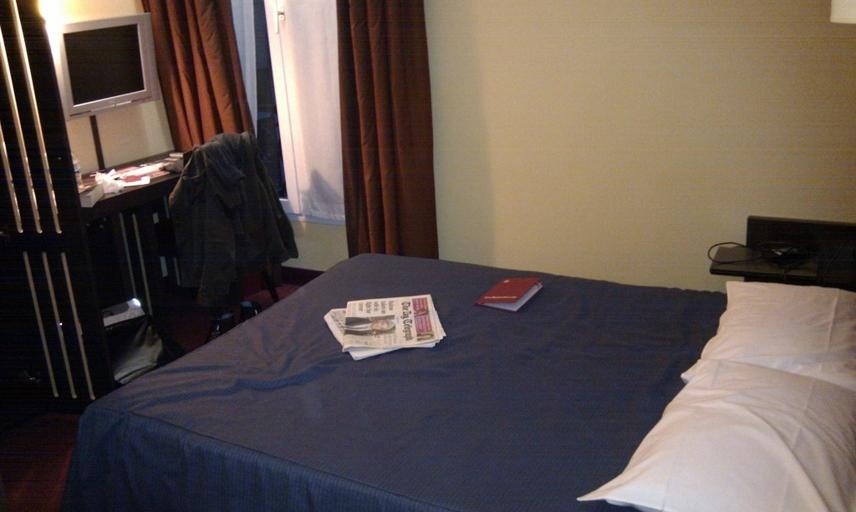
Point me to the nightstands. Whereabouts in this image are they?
[703,215,855,291]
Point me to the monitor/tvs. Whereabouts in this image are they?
[59,12,161,120]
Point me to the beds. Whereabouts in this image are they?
[55,251,856,510]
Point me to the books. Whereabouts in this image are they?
[474,277,545,312]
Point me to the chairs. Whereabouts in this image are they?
[146,129,293,365]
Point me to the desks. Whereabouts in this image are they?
[76,149,187,217]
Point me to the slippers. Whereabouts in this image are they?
[208,310,235,343]
[240,300,262,321]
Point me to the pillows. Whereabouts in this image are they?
[575,357,856,509]
[677,278,855,385]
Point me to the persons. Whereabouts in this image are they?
[345,315,397,336]
